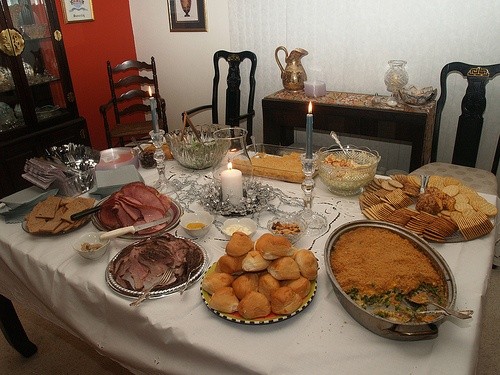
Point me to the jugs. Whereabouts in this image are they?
[274,46,309,93]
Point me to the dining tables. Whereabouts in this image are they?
[0,134,497,374]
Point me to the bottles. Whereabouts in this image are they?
[211,127,255,199]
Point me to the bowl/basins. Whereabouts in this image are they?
[314,143,382,197]
[166,124,232,170]
[267,214,309,246]
[72,231,110,260]
[221,217,258,241]
[180,211,214,237]
[228,142,319,184]
[0,185,61,210]
[399,86,438,107]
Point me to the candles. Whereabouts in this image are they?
[148,85,160,139]
[218,161,243,206]
[302,101,314,170]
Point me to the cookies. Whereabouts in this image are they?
[359,174,498,242]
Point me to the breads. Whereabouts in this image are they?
[203,232,318,320]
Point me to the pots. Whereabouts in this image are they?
[323,219,458,342]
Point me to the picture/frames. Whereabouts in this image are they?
[167,1,209,33]
[60,0,96,24]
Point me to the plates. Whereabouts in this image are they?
[90,192,182,241]
[104,233,209,301]
[200,258,317,326]
[302,79,327,98]
[21,213,92,237]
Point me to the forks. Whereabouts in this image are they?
[416,174,425,197]
[129,267,173,307]
[406,289,472,319]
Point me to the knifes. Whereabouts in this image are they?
[101,215,171,241]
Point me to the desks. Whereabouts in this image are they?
[262,86,438,175]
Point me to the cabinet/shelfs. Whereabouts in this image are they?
[0,1,93,200]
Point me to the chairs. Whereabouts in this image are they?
[429,62,499,181]
[182,48,258,149]
[97,58,170,149]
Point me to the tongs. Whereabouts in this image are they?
[179,111,206,148]
